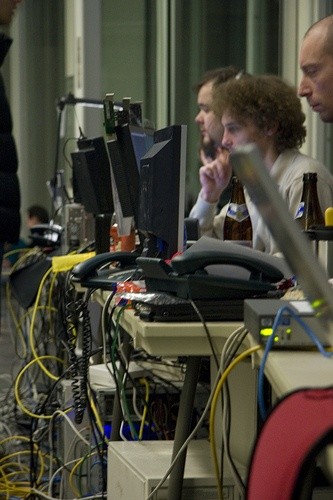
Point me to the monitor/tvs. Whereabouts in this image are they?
[70,123,188,275]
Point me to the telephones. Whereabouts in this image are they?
[70,253,152,291]
[145,249,287,298]
[27,225,64,249]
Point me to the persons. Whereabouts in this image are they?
[26,205,50,231]
[184,15,333,301]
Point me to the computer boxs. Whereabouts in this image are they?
[57,377,105,500]
[108,440,249,500]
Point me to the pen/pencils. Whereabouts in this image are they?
[218,147,226,160]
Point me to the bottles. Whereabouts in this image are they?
[223,176,253,241]
[293,172,326,234]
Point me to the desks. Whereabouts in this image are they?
[86,282,250,500]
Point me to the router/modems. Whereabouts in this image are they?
[90,359,153,388]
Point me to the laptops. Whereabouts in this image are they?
[228,143,333,319]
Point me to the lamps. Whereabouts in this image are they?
[67,90,126,115]
